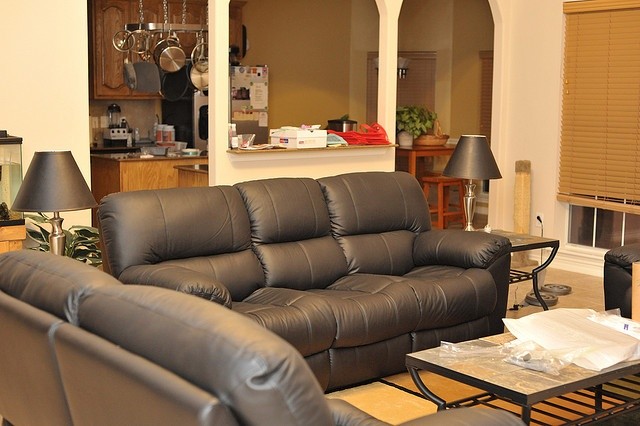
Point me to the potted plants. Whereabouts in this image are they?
[396,105,437,149]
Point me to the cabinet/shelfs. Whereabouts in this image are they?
[87,0,247,101]
[90,153,209,224]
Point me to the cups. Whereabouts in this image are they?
[232,136,237,148]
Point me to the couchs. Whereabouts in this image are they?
[0,246,527,426]
[99,171,511,395]
[604,242,640,319]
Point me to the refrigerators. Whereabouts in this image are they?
[230,66,269,144]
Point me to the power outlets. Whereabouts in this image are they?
[535,214,543,228]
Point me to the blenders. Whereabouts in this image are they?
[103,103,127,146]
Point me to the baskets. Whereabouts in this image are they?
[414,120,450,145]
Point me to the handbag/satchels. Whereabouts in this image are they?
[326,121,391,145]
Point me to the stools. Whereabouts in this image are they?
[421,177,466,229]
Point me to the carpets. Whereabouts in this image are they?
[581,405,640,426]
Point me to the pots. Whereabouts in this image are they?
[153,37,186,73]
[190,61,209,91]
[327,119,357,133]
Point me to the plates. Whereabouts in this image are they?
[240,148,263,150]
[160,71,189,102]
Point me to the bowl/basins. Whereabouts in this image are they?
[182,149,198,158]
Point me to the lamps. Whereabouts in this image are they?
[374,57,409,80]
[11,150,98,255]
[442,135,503,231]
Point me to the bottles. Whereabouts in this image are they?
[157,125,167,141]
[127,133,132,147]
[163,126,175,141]
[153,123,157,141]
[231,46,240,65]
[134,128,139,142]
[238,134,243,148]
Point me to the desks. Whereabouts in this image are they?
[397,145,457,178]
[489,230,558,311]
